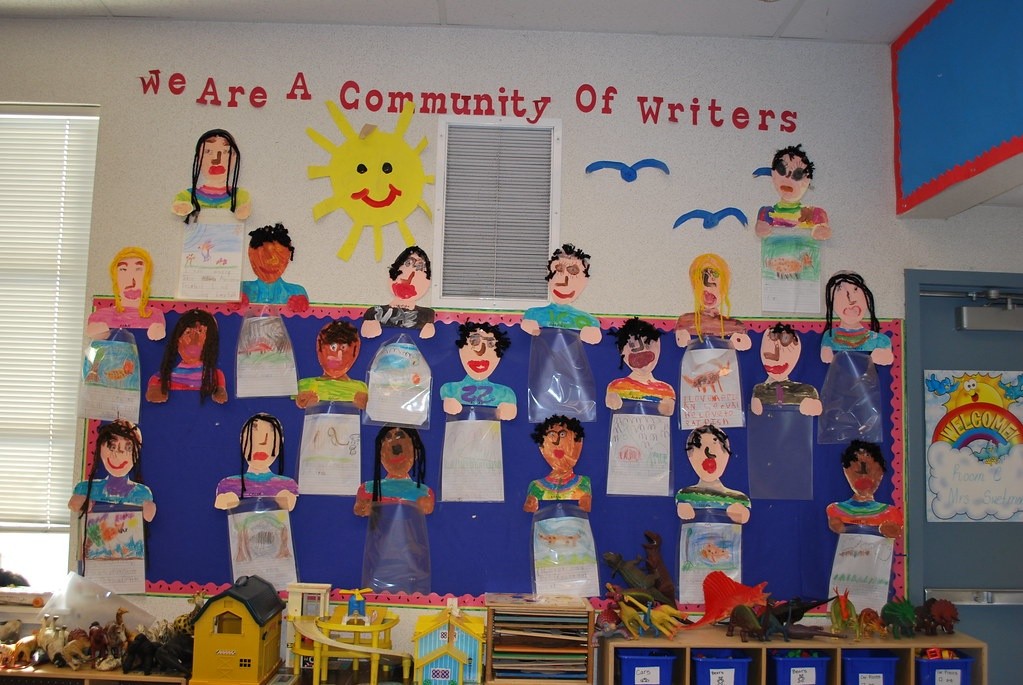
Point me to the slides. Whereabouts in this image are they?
[289,611,414,666]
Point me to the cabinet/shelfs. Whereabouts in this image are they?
[485,606,595,685]
[597,623,988,685]
[1,662,188,685]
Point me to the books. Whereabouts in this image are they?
[486,592,591,682]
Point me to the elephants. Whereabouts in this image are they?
[155,635,194,680]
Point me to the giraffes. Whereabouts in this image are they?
[174,590,205,638]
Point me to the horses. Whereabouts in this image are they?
[88,621,107,668]
[106,621,127,660]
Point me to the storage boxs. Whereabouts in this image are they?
[772,651,832,685]
[614,649,678,685]
[691,650,752,685]
[915,650,975,685]
[842,650,900,685]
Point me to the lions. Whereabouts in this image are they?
[62,628,92,670]
